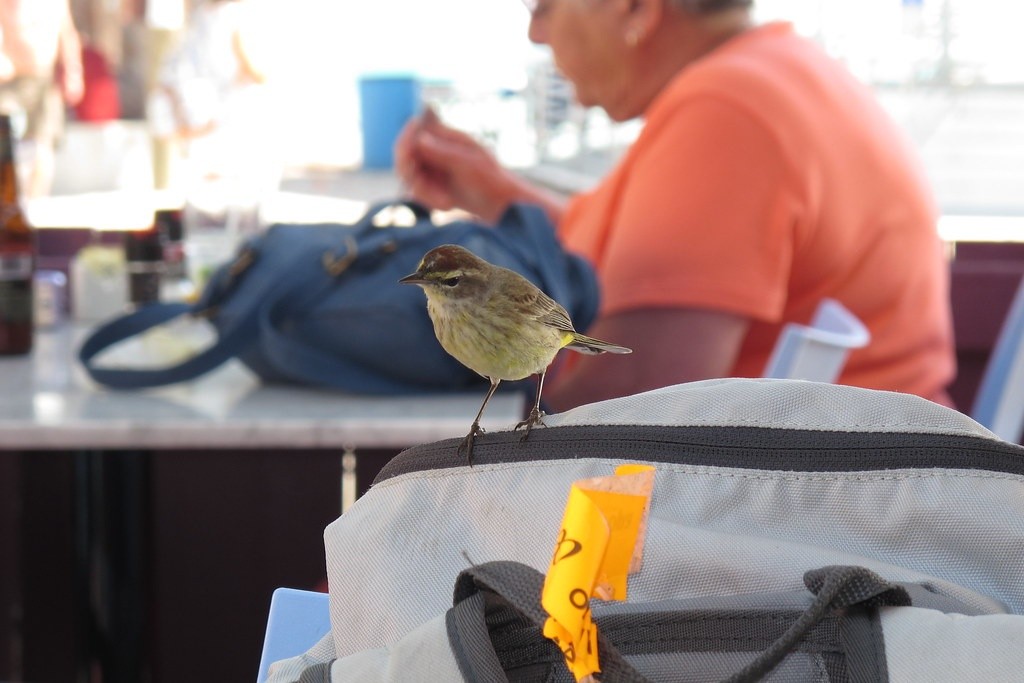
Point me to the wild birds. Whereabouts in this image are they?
[397,242,633,469]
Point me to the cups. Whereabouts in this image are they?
[360,76,420,172]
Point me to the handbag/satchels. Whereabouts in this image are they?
[265,374,1024,682]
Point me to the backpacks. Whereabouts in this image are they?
[81,195,595,397]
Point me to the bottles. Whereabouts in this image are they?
[0,113,34,355]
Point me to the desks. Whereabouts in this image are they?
[25,193,221,302]
[0,301,524,683]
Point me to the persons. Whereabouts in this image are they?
[395,1,958,406]
[0,2,264,192]
